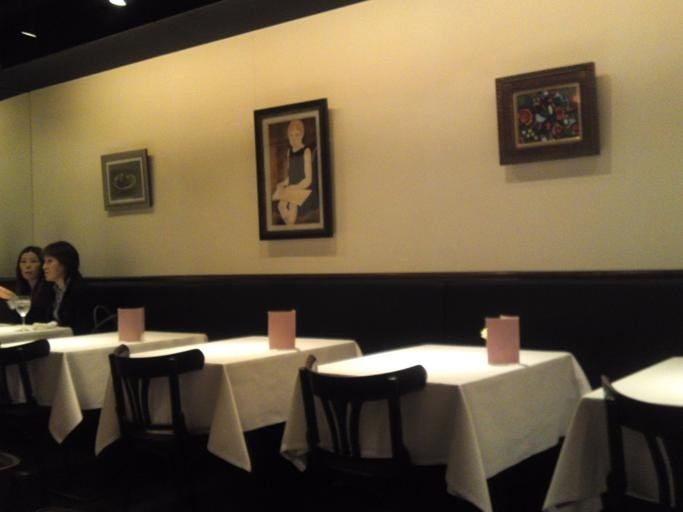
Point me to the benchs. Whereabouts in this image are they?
[82,274,259,338]
[255,269,447,354]
[447,267,682,383]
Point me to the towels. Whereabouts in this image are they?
[32,321,57,329]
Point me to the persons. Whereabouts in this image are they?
[17,246,43,324]
[276,120,313,226]
[0,240,94,335]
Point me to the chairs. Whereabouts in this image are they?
[299,366,442,508]
[1,341,92,484]
[110,346,205,484]
[598,374,683,512]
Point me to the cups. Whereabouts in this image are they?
[15,295,30,331]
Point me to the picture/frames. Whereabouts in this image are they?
[255,98,334,240]
[100,148,151,211]
[495,61,600,167]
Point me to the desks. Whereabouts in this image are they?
[317,344,569,508]
[584,357,683,512]
[2,319,67,339]
[1,331,207,452]
[131,336,356,476]
